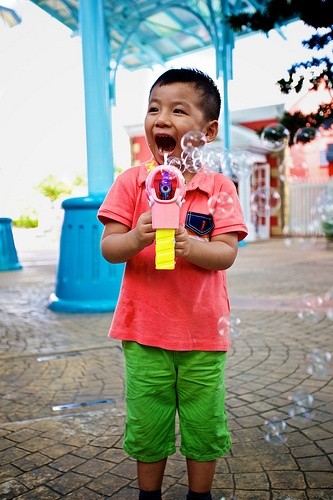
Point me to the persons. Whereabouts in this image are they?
[95,66,249,500]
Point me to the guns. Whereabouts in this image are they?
[145,151,188,271]
